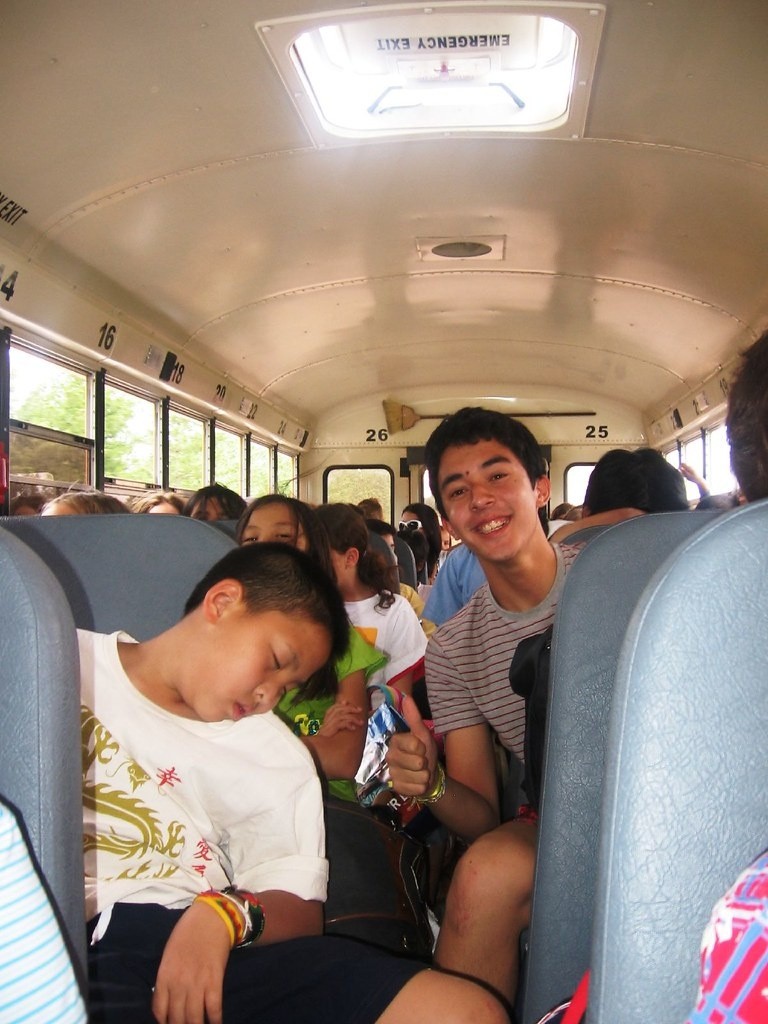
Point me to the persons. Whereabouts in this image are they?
[0,331,768,1024]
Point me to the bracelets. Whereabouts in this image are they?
[218,892,253,939]
[418,764,446,803]
[222,885,265,948]
[195,892,244,944]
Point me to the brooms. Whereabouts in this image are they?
[381,399,598,436]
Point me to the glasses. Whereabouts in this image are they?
[398,519,423,531]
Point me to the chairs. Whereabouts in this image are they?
[0,497,766,1022]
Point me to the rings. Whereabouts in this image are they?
[387,780,394,789]
